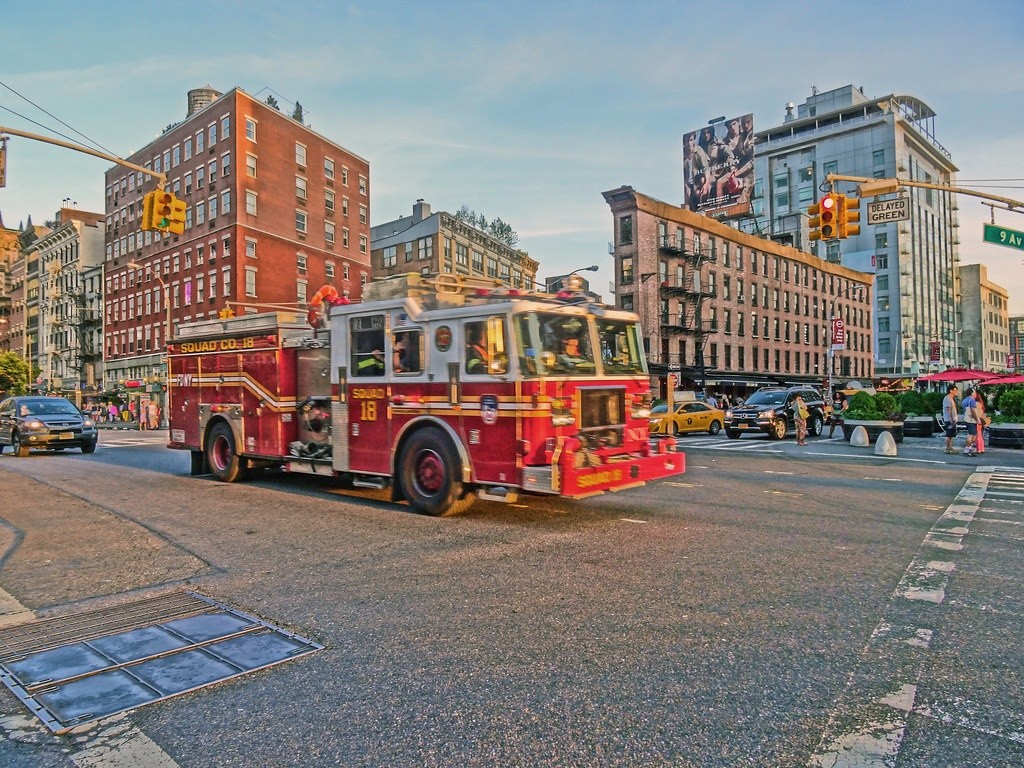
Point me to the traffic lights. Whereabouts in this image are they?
[839,193,860,240]
[142,189,187,235]
[808,194,838,242]
[162,385,166,392]
[219,310,236,319]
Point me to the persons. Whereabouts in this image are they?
[943,384,958,455]
[722,395,743,411]
[827,392,847,438]
[85,400,136,424]
[21,404,35,415]
[706,394,718,408]
[557,333,588,365]
[140,401,147,430]
[149,401,161,429]
[790,394,809,445]
[961,388,986,457]
[650,397,657,405]
[358,330,490,377]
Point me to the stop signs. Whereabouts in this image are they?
[668,373,679,388]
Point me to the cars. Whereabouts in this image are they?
[648,402,726,435]
[0,396,98,457]
[825,380,876,425]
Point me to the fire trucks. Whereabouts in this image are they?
[167,270,687,514]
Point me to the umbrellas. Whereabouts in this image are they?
[916,368,1024,393]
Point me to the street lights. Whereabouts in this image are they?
[127,261,171,340]
[498,261,599,293]
[928,330,963,392]
[83,264,105,392]
[829,284,865,402]
[7,299,32,395]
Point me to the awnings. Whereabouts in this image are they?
[682,373,779,388]
[778,378,823,385]
[107,386,146,396]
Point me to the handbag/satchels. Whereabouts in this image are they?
[798,401,809,419]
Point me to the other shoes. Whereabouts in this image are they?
[962,450,976,457]
[973,450,985,454]
[796,441,808,446]
[944,448,958,455]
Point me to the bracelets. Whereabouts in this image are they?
[393,350,401,353]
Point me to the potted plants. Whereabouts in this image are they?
[922,392,955,433]
[895,391,933,437]
[989,389,1024,449]
[844,391,904,443]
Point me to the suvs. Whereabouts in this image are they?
[724,387,827,440]
